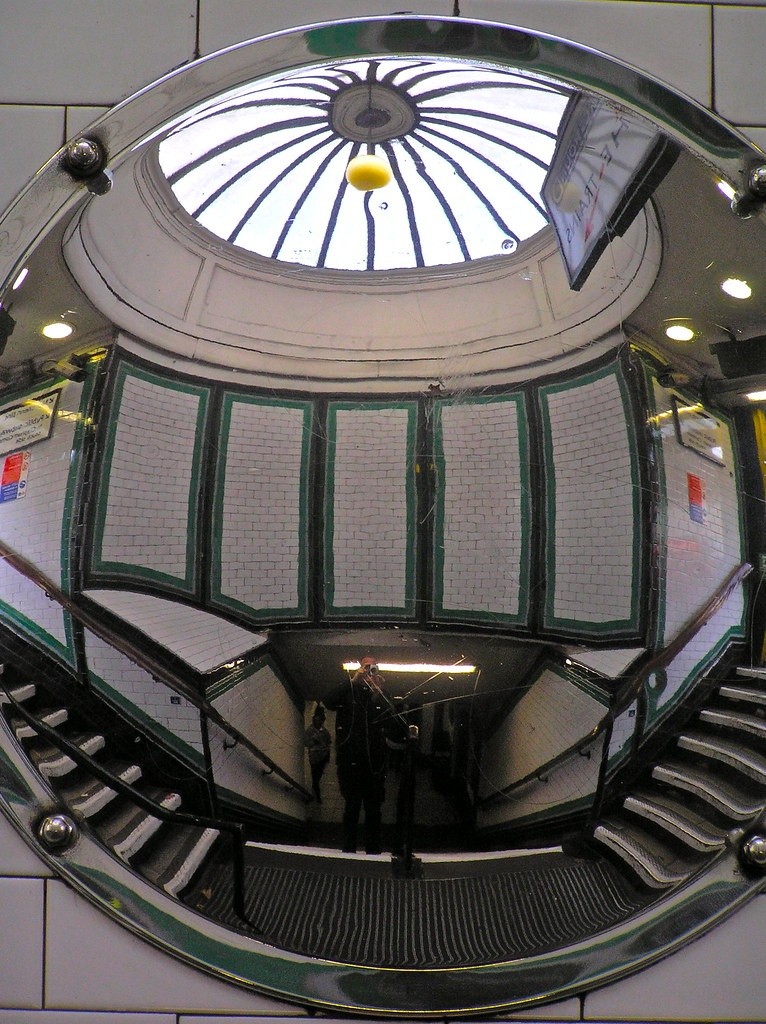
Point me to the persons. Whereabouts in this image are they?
[321,654,419,853]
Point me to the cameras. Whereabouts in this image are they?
[365,664,379,677]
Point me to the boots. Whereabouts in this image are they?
[310,779,323,803]
